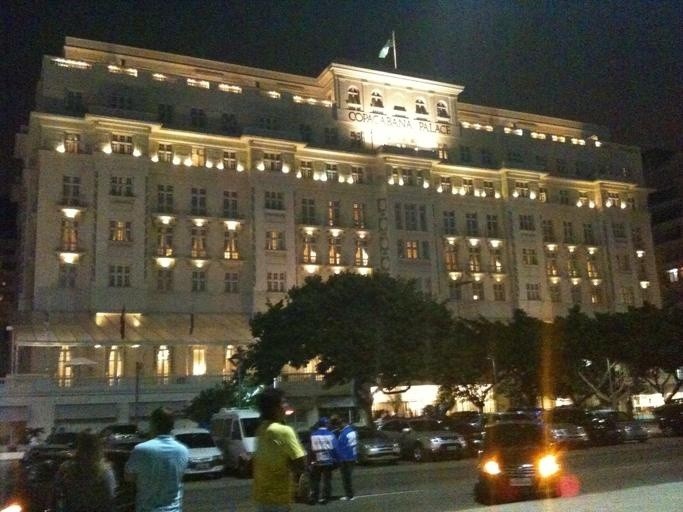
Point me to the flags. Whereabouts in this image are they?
[378,36,393,59]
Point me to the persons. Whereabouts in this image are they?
[308,418,337,504]
[50,428,117,511]
[336,418,358,501]
[122,405,193,512]
[29,427,64,450]
[252,388,305,512]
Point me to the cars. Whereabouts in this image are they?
[383,404,648,461]
[15,404,310,510]
[478,421,562,500]
[349,427,401,467]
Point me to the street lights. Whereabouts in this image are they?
[482,354,500,411]
[575,355,592,404]
[133,362,143,420]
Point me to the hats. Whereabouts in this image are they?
[319,416,329,426]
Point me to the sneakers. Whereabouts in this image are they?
[318,498,328,504]
[339,494,355,501]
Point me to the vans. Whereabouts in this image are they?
[654,396,683,438]
